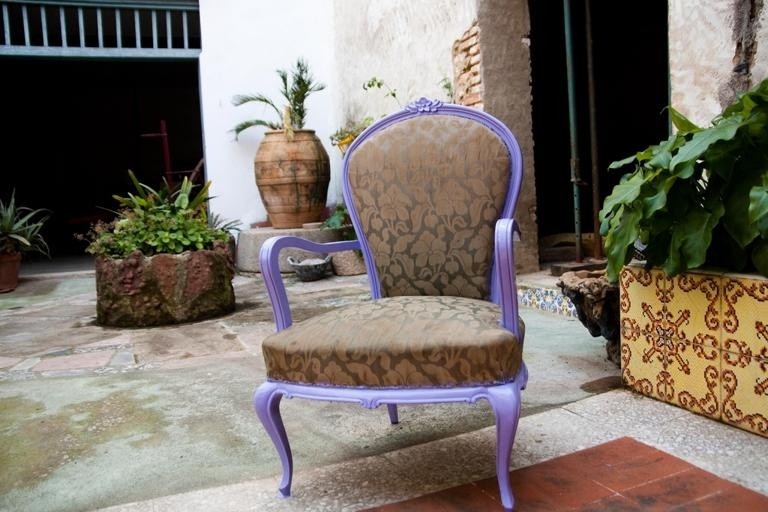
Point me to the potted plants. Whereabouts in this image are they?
[72,167,244,326]
[601,77,768,437]
[225,56,331,229]
[0,192,48,294]
[329,128,356,156]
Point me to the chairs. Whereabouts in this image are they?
[253,98,526,511]
